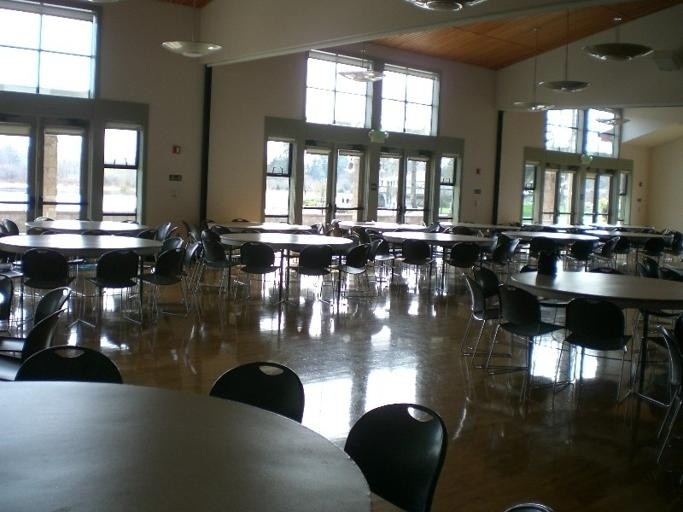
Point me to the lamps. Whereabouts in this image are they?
[161,0,221,58]
[339,42,385,82]
[581,13,654,63]
[513,27,557,113]
[579,152,593,166]
[406,0,486,13]
[540,6,591,93]
[368,130,388,144]
